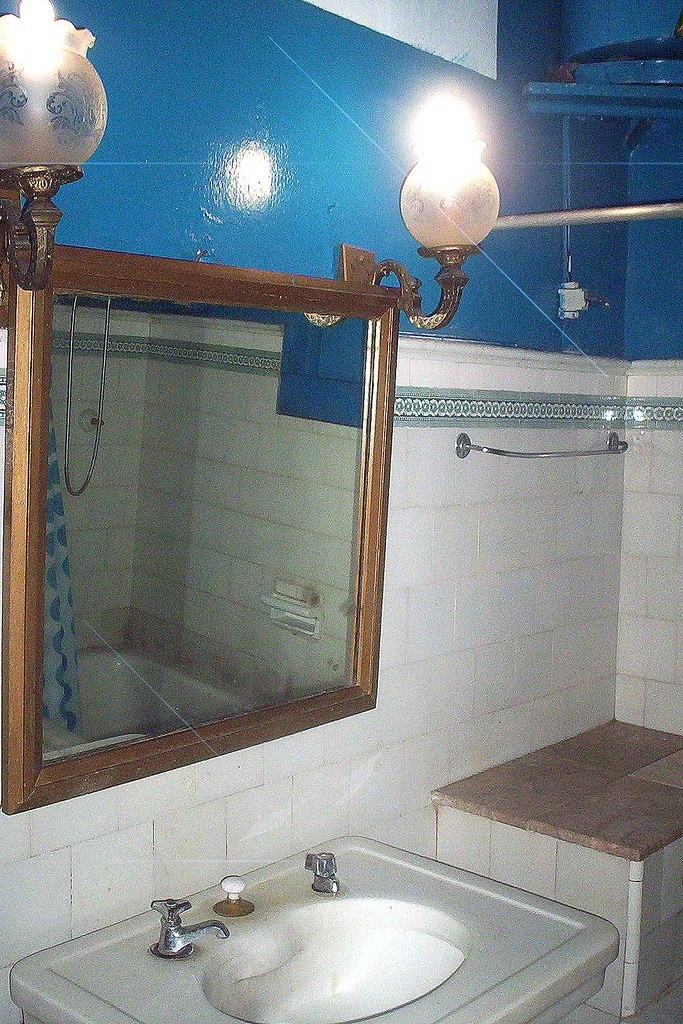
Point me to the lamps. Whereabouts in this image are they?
[0,1,108,291]
[340,96,500,335]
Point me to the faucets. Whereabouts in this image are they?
[146,896,231,960]
[304,850,342,895]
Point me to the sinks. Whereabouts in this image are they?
[195,886,475,1024]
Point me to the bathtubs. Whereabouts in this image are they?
[41,641,249,755]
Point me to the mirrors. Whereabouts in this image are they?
[0,240,402,818]
